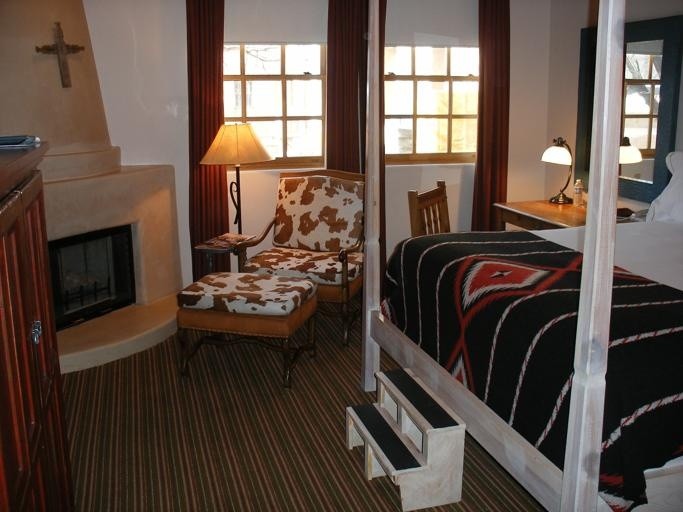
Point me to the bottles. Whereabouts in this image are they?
[573,179,583,209]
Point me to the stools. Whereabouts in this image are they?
[176,270,317,388]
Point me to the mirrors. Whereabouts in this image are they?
[571,15,683,203]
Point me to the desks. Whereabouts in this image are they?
[495,195,639,231]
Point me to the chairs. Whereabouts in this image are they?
[408,180,451,239]
[234,169,364,346]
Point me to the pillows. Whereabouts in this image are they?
[645,152,683,224]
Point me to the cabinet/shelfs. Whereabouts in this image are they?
[0,143,74,512]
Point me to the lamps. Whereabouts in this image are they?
[200,123,276,233]
[541,136,575,203]
[618,137,642,163]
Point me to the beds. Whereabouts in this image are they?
[362,217,683,512]
